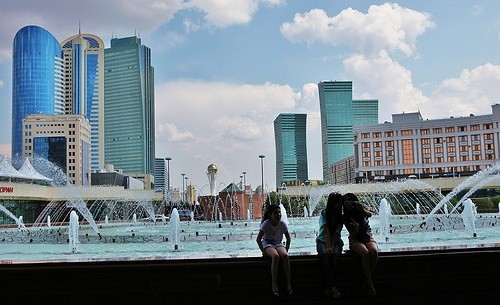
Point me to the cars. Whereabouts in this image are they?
[143,215,170,221]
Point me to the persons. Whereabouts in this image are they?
[316,192,343,297]
[342,193,378,297]
[257,205,292,296]
[372,180,404,183]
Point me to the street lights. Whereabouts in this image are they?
[180,173,186,206]
[240,170,246,212]
[259,155,266,216]
[164,156,172,192]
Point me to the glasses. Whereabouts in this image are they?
[333,190,340,197]
[343,201,354,205]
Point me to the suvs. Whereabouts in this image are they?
[178,210,191,220]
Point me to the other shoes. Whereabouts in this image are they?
[286,287,293,294]
[271,287,280,297]
[368,285,377,296]
[324,287,340,298]
